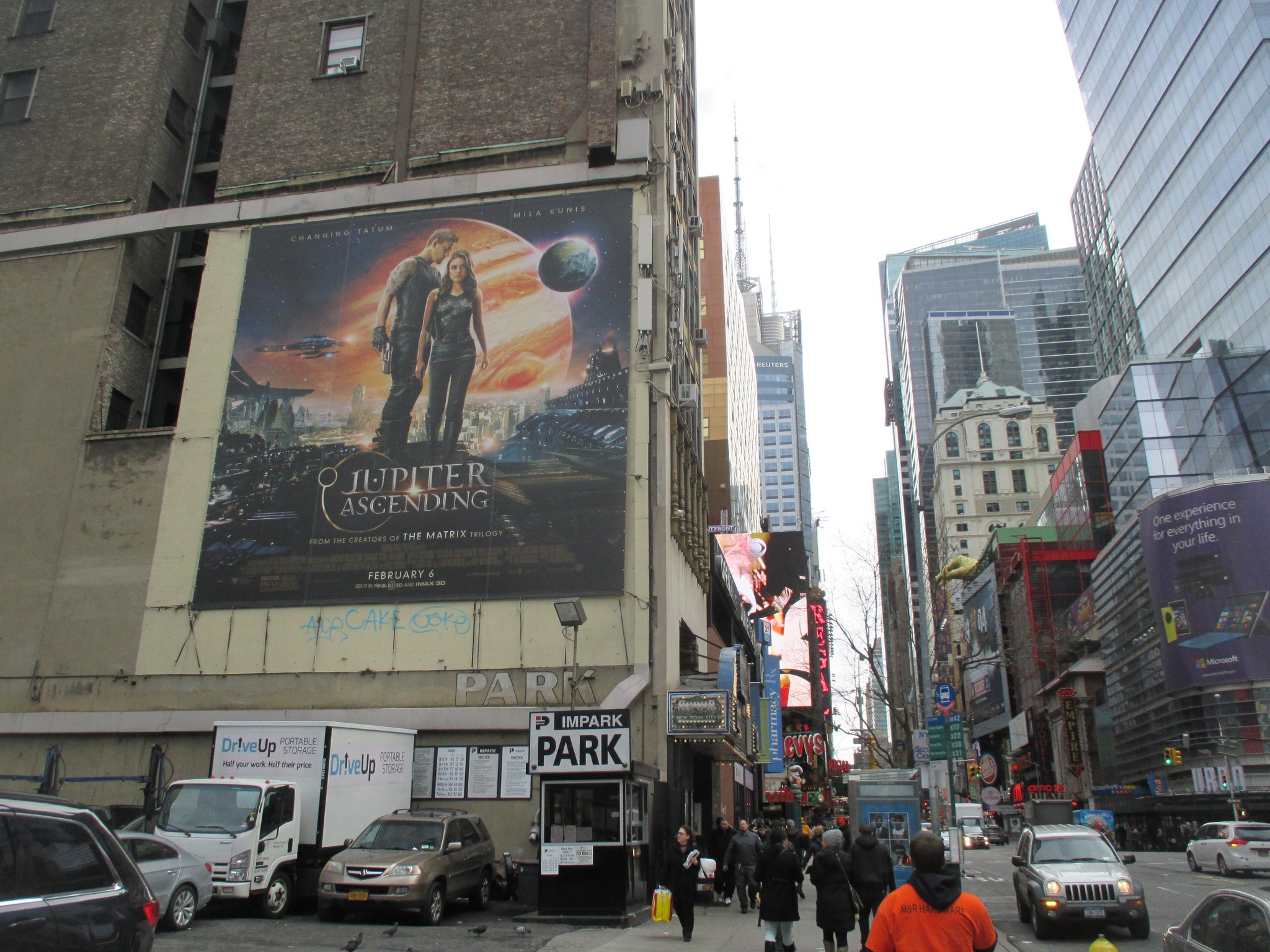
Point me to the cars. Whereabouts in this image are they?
[112,828,211,930]
[1161,882,1269,952]
[921,822,951,849]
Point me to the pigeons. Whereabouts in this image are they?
[339,922,531,952]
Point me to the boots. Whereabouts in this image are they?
[764,940,775,952]
[782,942,795,952]
[392,415,419,468]
[440,419,463,471]
[837,946,848,952]
[822,940,835,952]
[372,418,404,470]
[775,937,783,952]
[423,414,443,469]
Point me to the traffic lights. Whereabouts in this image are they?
[1171,748,1181,766]
[1221,774,1227,788]
[969,766,976,779]
[1164,748,1171,766]
[1237,809,1246,818]
[977,767,980,778]
[924,801,929,808]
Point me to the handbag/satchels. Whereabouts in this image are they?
[849,884,864,913]
[652,887,673,922]
[693,860,715,902]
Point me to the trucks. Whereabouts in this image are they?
[1022,799,1073,827]
[145,719,418,919]
[945,803,990,849]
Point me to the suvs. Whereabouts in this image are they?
[1011,820,1151,941]
[317,807,498,927]
[1,790,156,952]
[1186,820,1269,876]
[983,825,1004,847]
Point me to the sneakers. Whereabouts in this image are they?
[859,942,873,952]
[683,936,691,941]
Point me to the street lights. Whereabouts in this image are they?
[1006,757,1041,799]
[918,402,1034,833]
[790,785,803,831]
[1213,693,1240,820]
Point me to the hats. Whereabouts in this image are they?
[786,819,795,830]
[757,823,766,829]
[836,818,845,824]
[768,826,788,843]
[813,828,822,838]
[858,824,873,835]
[822,830,843,848]
[715,815,723,828]
[800,817,804,821]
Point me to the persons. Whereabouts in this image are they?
[752,824,805,952]
[659,824,702,943]
[373,228,458,469]
[850,825,897,952]
[707,800,909,913]
[810,828,859,952]
[1101,818,1193,852]
[408,249,490,472]
[862,830,999,952]
